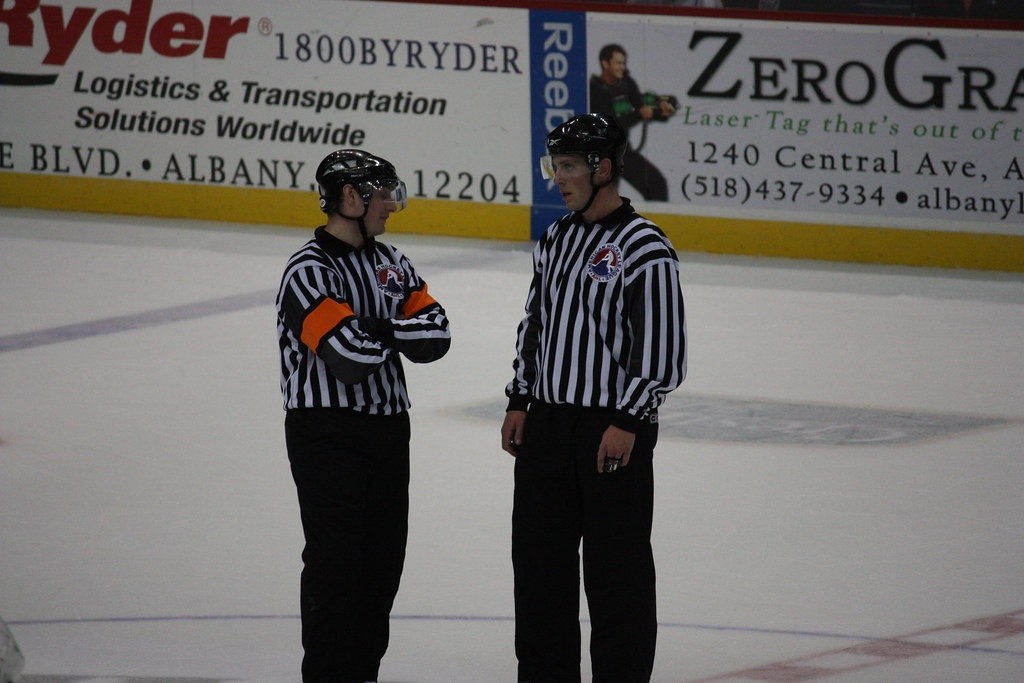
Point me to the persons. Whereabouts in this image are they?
[275,149,451,683]
[588,43,676,204]
[500,112,688,683]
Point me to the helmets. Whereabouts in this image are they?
[547,112,627,169]
[314,148,401,215]
